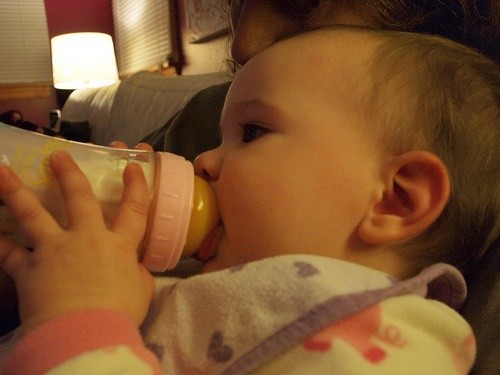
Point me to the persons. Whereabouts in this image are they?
[0,0,500,375]
[0,26,500,375]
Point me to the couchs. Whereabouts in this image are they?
[61,70,232,154]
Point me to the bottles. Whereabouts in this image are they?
[0,122,220,272]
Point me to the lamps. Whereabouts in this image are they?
[51,32,119,90]
